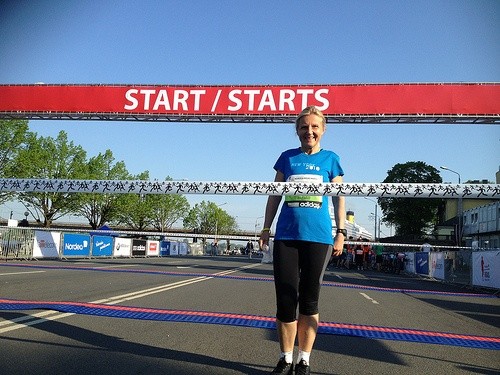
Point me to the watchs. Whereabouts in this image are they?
[336,229,347,236]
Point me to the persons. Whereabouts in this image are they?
[327,238,407,274]
[258,106,348,375]
[245,239,254,260]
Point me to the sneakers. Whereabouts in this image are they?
[294,358,311,375]
[268,355,294,375]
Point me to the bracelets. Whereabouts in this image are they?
[261,228,271,234]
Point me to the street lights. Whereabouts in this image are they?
[364,197,377,242]
[255,216,265,242]
[439,165,463,247]
[370,212,380,242]
